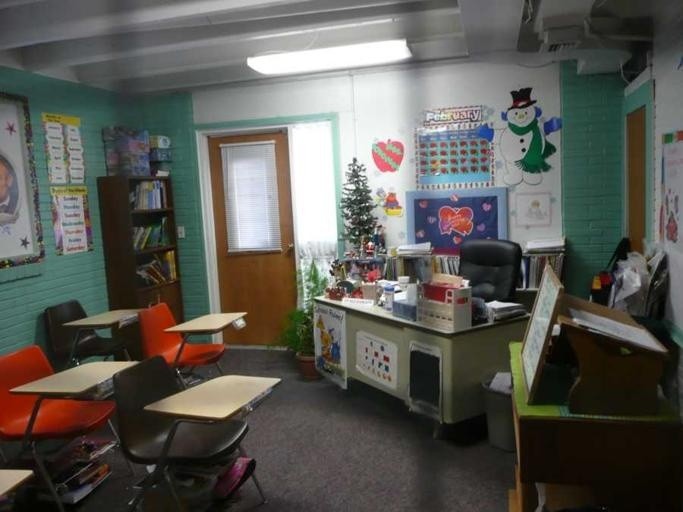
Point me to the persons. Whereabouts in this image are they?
[0,159,18,215]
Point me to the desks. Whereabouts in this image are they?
[1,468,32,497]
[10,359,139,398]
[63,310,144,328]
[142,375,283,422]
[163,311,247,336]
[311,289,530,444]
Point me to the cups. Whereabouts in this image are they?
[383,283,395,313]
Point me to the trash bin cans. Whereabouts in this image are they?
[480,377,516,452]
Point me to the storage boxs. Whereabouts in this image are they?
[101,126,172,176]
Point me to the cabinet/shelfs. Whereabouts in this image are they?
[507,340,683,512]
[95,174,184,361]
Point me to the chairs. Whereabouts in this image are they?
[455,238,522,302]
[113,355,268,510]
[44,299,113,365]
[3,346,133,508]
[138,303,226,388]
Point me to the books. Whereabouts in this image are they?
[131,169,178,288]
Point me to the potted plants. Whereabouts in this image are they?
[263,257,331,381]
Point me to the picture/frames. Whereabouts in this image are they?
[404,186,508,252]
[520,263,565,405]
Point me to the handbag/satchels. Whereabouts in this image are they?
[590,238,630,305]
